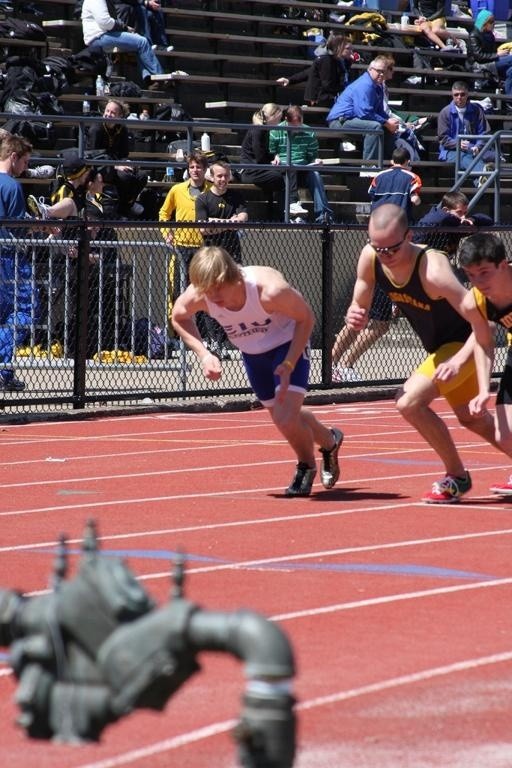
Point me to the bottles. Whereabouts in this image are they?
[95,75,105,97]
[139,110,150,120]
[445,35,453,47]
[166,158,175,182]
[201,132,210,152]
[400,13,410,31]
[83,93,92,112]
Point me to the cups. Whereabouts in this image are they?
[462,141,470,152]
[175,149,184,162]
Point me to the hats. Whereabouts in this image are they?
[475,10,493,32]
[62,156,87,180]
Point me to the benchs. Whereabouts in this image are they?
[0,1,510,209]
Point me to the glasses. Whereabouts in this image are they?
[454,93,466,97]
[373,69,386,74]
[367,229,410,257]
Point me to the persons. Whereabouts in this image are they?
[81,1,174,91]
[27,157,124,358]
[171,244,345,494]
[437,82,498,190]
[242,103,335,225]
[469,10,511,94]
[276,35,423,178]
[459,233,512,498]
[410,1,461,53]
[346,203,505,504]
[367,148,421,227]
[1,129,61,392]
[332,191,494,383]
[158,152,248,362]
[84,100,148,214]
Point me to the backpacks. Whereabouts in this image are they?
[194,147,229,165]
[1,0,79,149]
[164,139,199,182]
[155,104,193,143]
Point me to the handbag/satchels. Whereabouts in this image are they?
[105,81,142,98]
[131,317,174,361]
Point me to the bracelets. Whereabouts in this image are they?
[282,360,296,371]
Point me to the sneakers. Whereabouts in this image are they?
[318,428,343,489]
[404,77,428,85]
[478,162,493,189]
[360,165,381,177]
[0,374,25,391]
[488,475,512,496]
[285,462,317,495]
[283,201,309,216]
[339,141,357,153]
[420,468,473,504]
[129,203,144,215]
